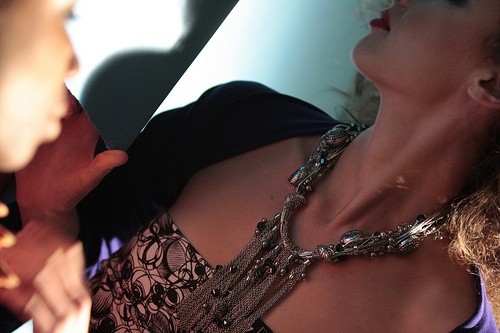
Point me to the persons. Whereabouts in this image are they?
[12,0,500,333]
[1,1,91,333]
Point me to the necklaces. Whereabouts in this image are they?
[175,116,470,333]
